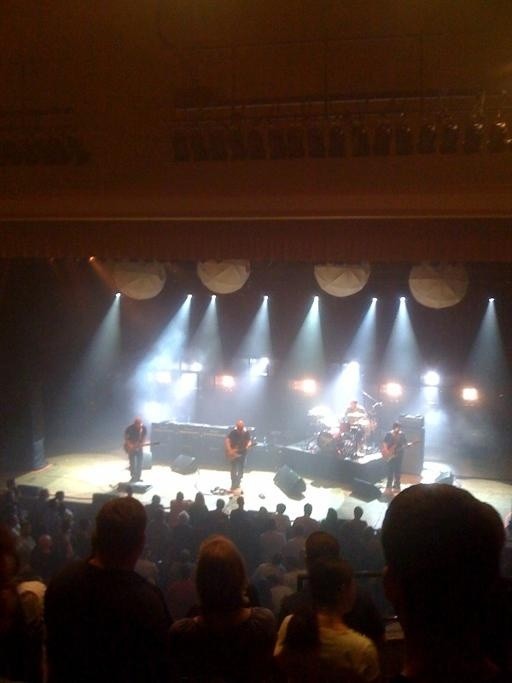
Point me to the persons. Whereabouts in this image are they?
[381,421,412,489]
[223,419,251,491]
[340,399,368,451]
[2,476,512,683]
[123,415,147,482]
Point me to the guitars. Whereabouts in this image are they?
[227,441,257,460]
[124,438,160,452]
[381,439,419,462]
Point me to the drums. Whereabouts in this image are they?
[317,430,342,450]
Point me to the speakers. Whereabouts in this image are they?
[171,453,200,474]
[436,470,454,485]
[142,452,152,468]
[274,466,308,495]
[350,477,381,500]
